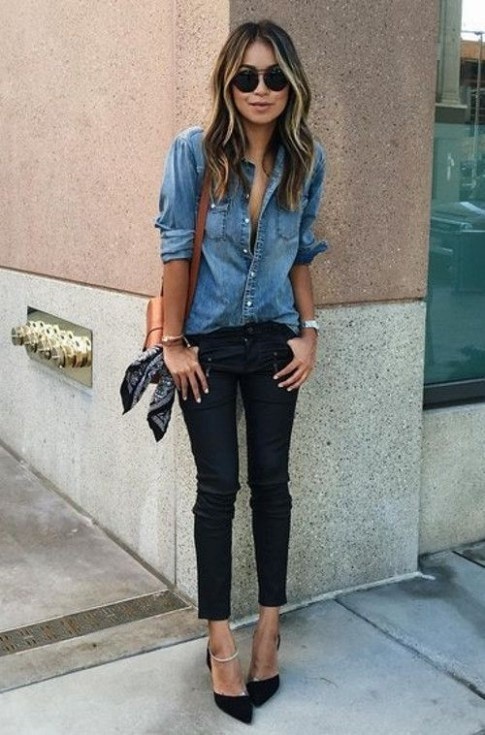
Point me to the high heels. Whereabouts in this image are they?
[246,632,280,707]
[206,641,252,725]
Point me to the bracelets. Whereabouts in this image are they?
[161,335,191,349]
[301,320,319,336]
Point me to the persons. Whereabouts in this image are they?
[155,19,328,724]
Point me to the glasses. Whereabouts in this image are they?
[230,68,289,93]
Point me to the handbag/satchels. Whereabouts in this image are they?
[142,296,163,383]
